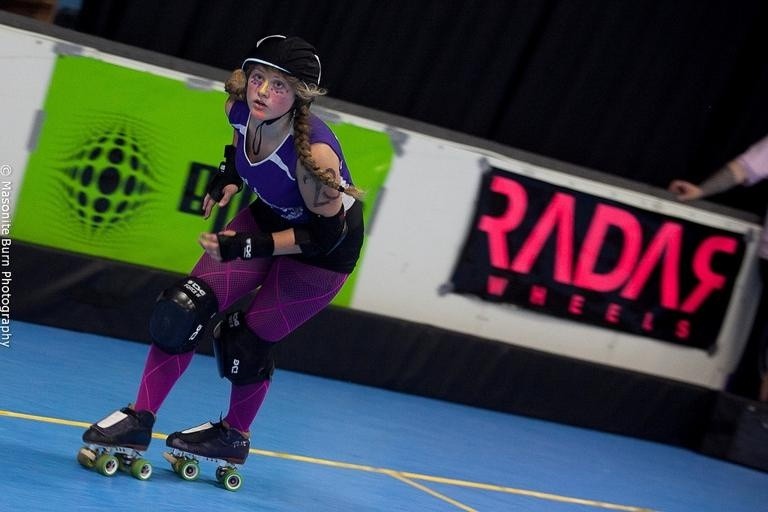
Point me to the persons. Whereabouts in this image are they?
[82,28,364,464]
[667,135,768,403]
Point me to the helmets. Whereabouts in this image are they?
[241,34,323,88]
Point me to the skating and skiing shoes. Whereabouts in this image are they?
[163,419,250,490]
[77,404,153,480]
